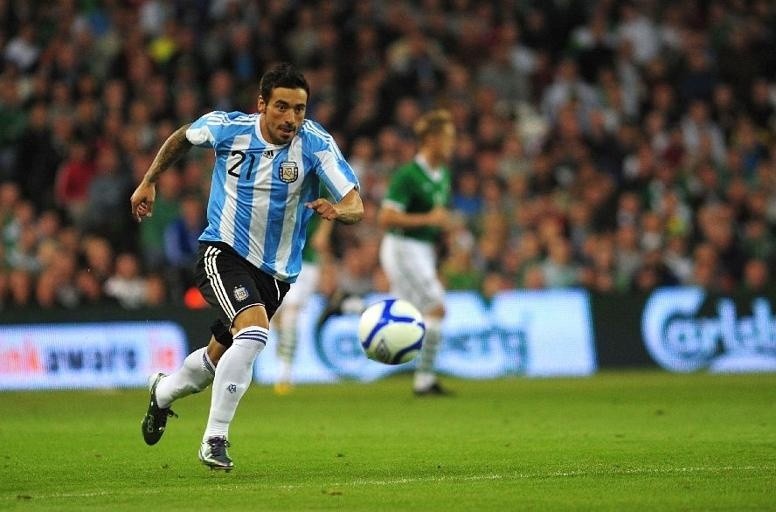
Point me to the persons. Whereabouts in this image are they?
[378,110,471,396]
[132,60,366,466]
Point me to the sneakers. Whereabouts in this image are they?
[410,377,456,397]
[196,434,235,472]
[140,371,182,446]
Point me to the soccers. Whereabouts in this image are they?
[357,299,426,364]
